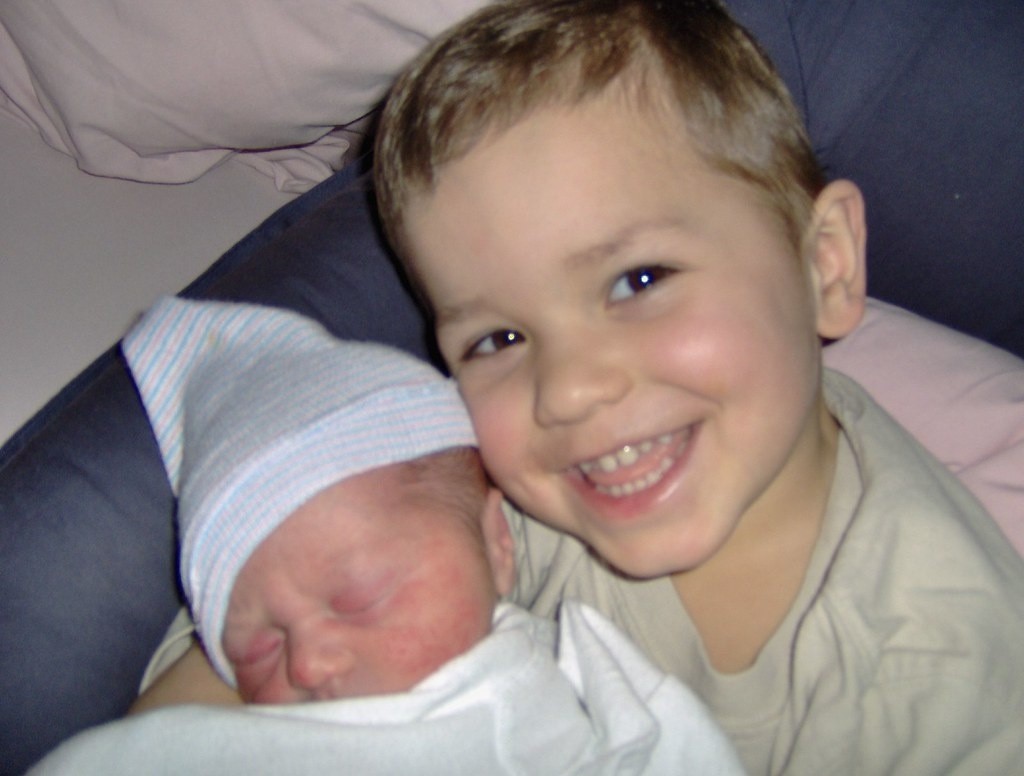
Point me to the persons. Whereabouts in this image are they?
[125,1,1023,775]
[18,296,746,773]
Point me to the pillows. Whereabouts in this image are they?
[0,0,489,183]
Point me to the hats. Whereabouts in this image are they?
[121,298,483,691]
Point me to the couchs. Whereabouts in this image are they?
[0,0,1024,776]
